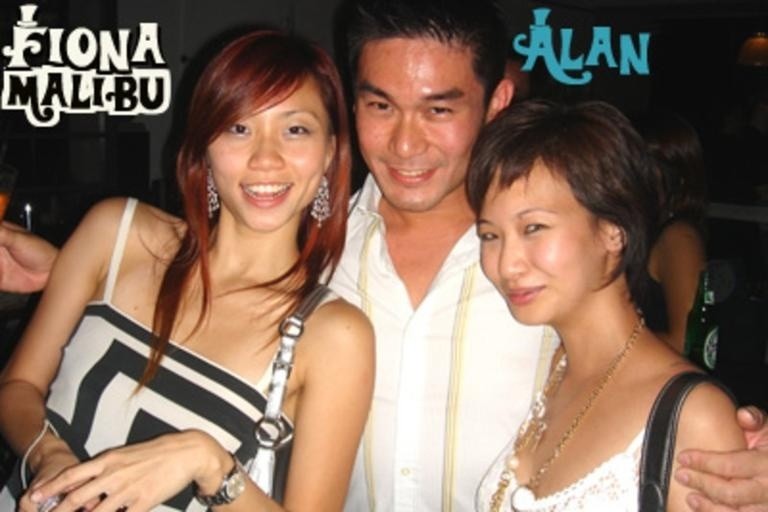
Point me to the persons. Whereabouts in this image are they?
[0,0,768,511]
[635,124,709,357]
[464,98,751,512]
[1,30,376,512]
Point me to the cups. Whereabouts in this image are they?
[0,163,18,221]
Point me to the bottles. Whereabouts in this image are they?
[682,270,719,375]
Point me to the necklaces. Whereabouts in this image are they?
[491,308,647,511]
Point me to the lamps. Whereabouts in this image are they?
[735,0,768,67]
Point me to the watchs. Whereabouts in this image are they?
[194,452,246,506]
